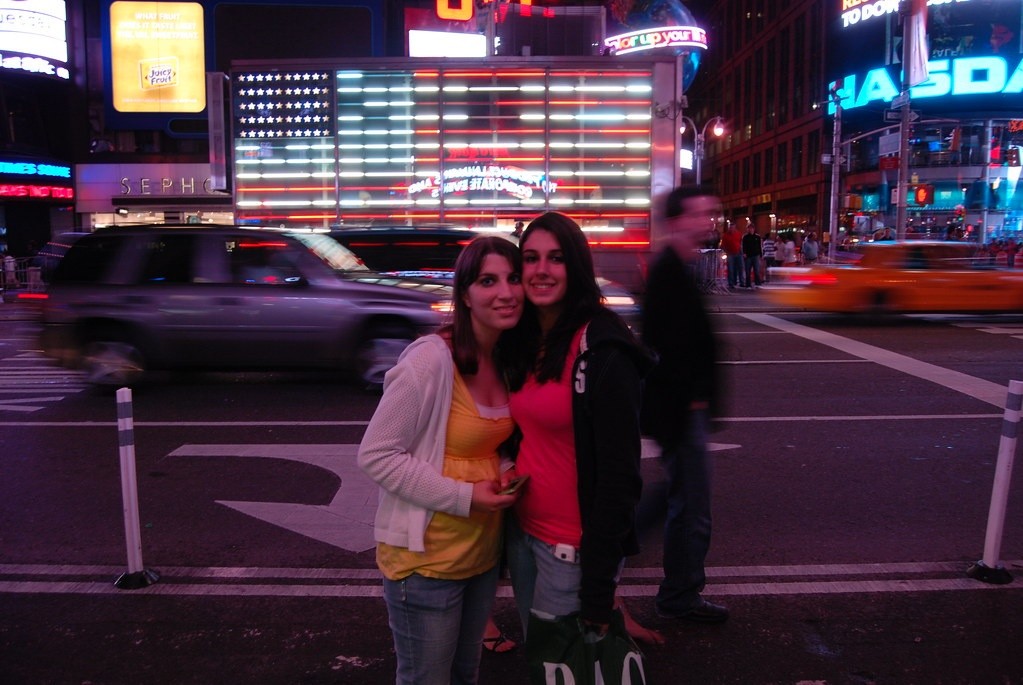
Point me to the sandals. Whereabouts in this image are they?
[480,616,518,653]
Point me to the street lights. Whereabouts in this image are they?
[681,115,726,188]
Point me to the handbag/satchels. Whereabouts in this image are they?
[525,608,651,685]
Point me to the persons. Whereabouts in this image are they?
[356,211,712,685]
[634,180,730,637]
[694,222,1023,291]
[0,239,45,291]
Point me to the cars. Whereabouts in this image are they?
[753,237,1022,321]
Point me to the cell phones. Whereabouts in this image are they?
[556,543,575,563]
[498,474,530,495]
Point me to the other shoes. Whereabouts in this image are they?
[587,596,666,647]
[729,284,736,289]
[740,284,746,288]
[655,595,729,622]
[747,285,752,290]
[755,284,763,288]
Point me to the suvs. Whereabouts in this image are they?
[35,220,455,400]
